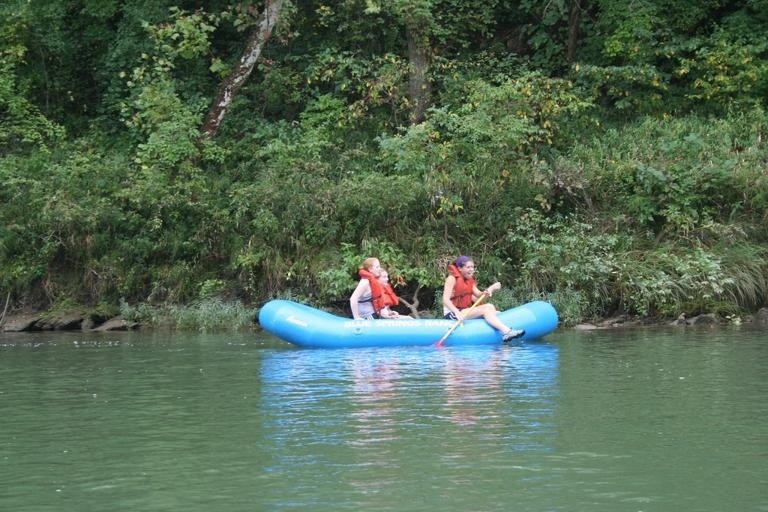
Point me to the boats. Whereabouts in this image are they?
[258,300,558,345]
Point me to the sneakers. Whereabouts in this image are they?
[502,328,526,342]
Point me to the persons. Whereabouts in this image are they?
[447,350,503,429]
[350,351,394,455]
[372,268,411,318]
[439,255,525,343]
[349,257,382,324]
